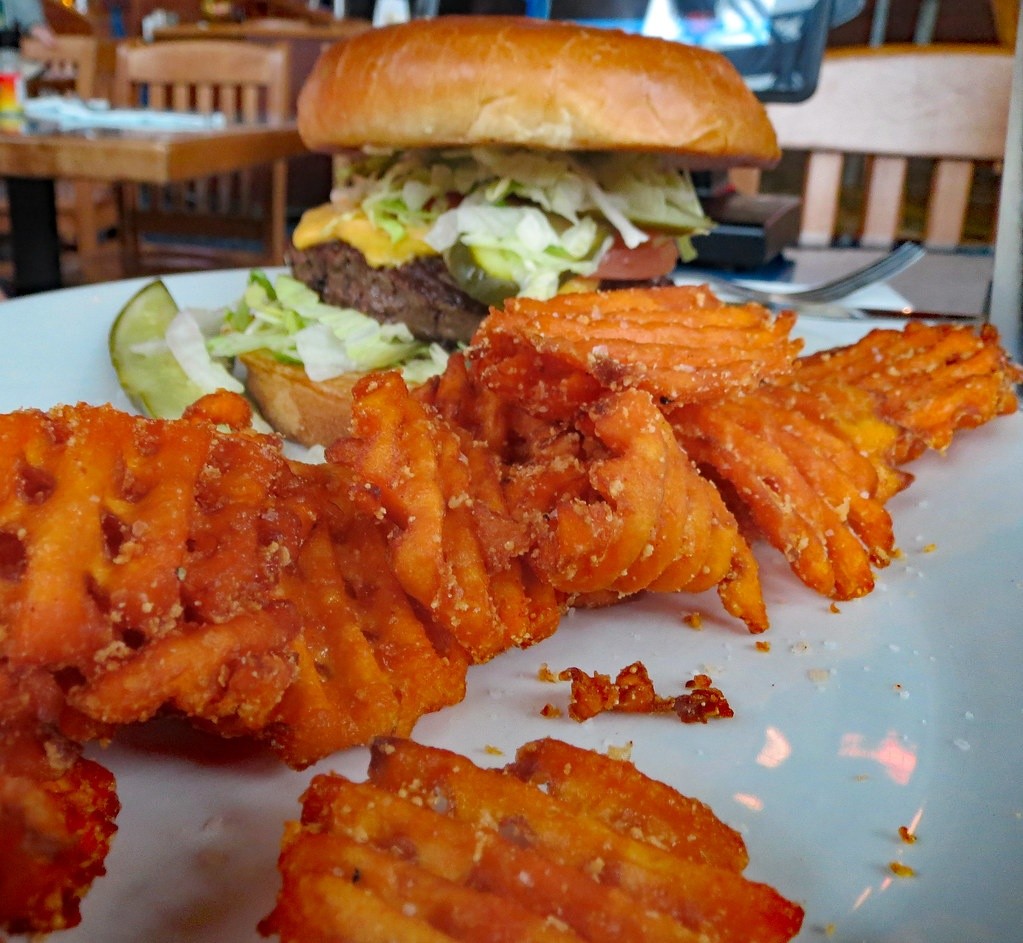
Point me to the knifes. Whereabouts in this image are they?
[719,283,976,323]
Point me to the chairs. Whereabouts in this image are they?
[22,18,295,281]
[729,44,1014,247]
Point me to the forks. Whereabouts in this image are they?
[782,238,927,303]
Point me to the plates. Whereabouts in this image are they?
[0,265,1022,943]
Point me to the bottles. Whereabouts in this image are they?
[0,30,25,137]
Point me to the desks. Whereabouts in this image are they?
[0,117,314,286]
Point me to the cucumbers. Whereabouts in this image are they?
[109,280,205,417]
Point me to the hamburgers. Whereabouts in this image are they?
[208,15,783,445]
